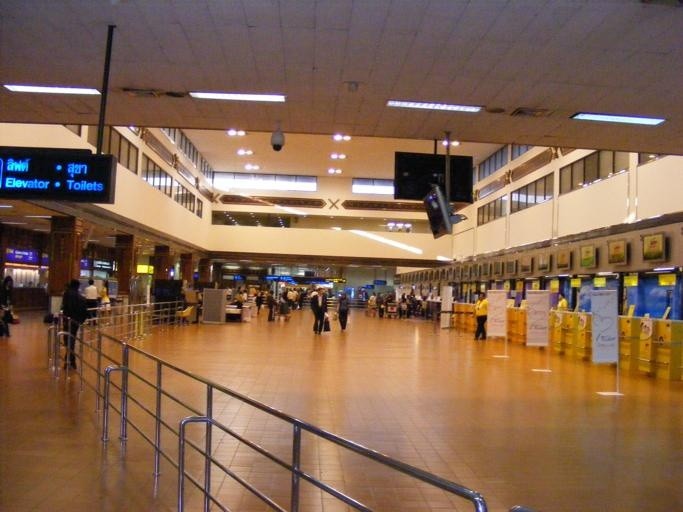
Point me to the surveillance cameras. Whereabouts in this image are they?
[271,134,284,151]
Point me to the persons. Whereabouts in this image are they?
[237,286,248,302]
[230,298,243,309]
[474,292,489,340]
[310,286,328,335]
[557,292,568,312]
[24,280,49,289]
[84,280,111,326]
[255,292,263,313]
[1,275,14,337]
[398,293,428,318]
[368,291,393,318]
[267,287,305,321]
[62,279,87,369]
[336,290,350,330]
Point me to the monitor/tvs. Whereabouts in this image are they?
[394,151,472,203]
[423,185,453,239]
